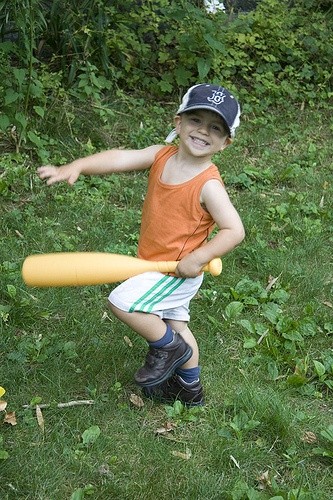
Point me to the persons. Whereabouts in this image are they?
[36,82,246,407]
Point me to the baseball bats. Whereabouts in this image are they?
[21,251,223,288]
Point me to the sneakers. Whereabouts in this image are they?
[135,329,193,386]
[144,374,204,407]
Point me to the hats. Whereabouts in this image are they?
[177,84,241,139]
